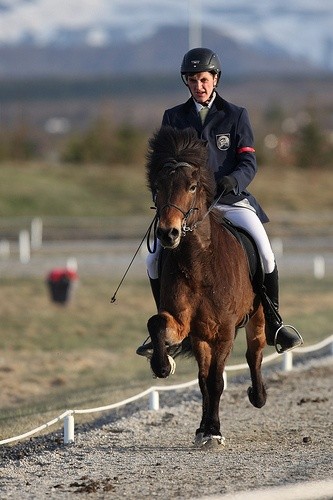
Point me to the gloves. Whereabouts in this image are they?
[216,175,238,196]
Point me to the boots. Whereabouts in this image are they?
[262,260,299,346]
[137,271,159,356]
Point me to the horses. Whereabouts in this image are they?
[133,122,269,454]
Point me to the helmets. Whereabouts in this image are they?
[180,48,220,74]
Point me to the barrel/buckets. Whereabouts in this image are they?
[48,274,78,304]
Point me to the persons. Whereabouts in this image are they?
[146,48,301,378]
